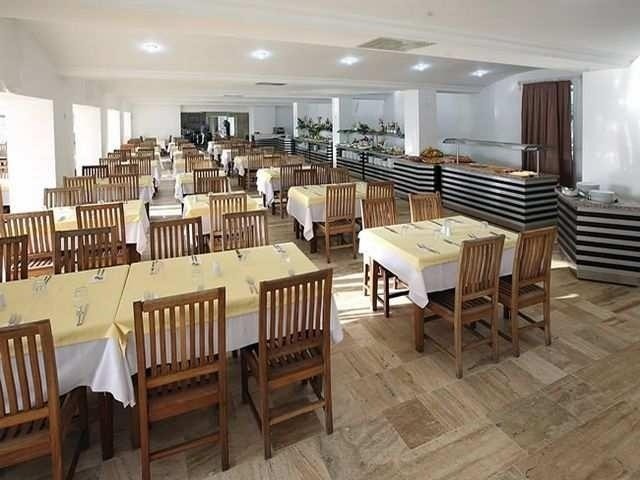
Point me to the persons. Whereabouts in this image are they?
[183,120,256,147]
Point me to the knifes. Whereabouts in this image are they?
[8,243,286,326]
[384,216,510,256]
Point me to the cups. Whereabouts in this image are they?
[341,151,393,168]
[285,256,296,275]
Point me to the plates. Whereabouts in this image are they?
[589,190,615,203]
[577,183,601,194]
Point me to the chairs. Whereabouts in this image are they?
[42,137,356,264]
[360,181,551,379]
[2,201,333,478]
